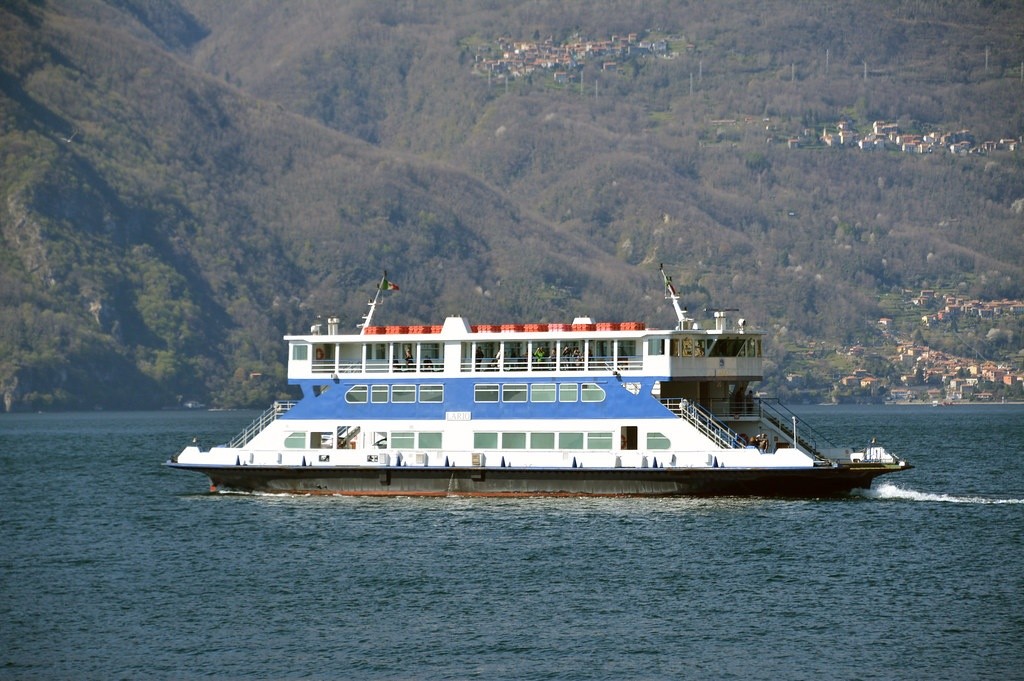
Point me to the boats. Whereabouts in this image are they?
[161,268,918,498]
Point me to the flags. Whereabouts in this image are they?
[381,279,399,291]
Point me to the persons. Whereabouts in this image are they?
[742,433,769,453]
[388,349,434,372]
[746,390,754,416]
[476,346,595,371]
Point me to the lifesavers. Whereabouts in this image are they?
[621,434,627,449]
[738,432,749,443]
[316,348,324,360]
